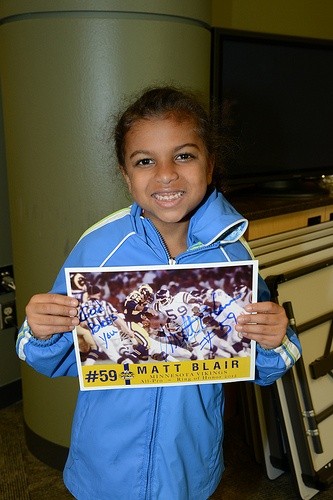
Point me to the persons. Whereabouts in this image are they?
[15,86,302,500]
[68,263,254,365]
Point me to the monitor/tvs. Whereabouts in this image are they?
[209,26,332,185]
